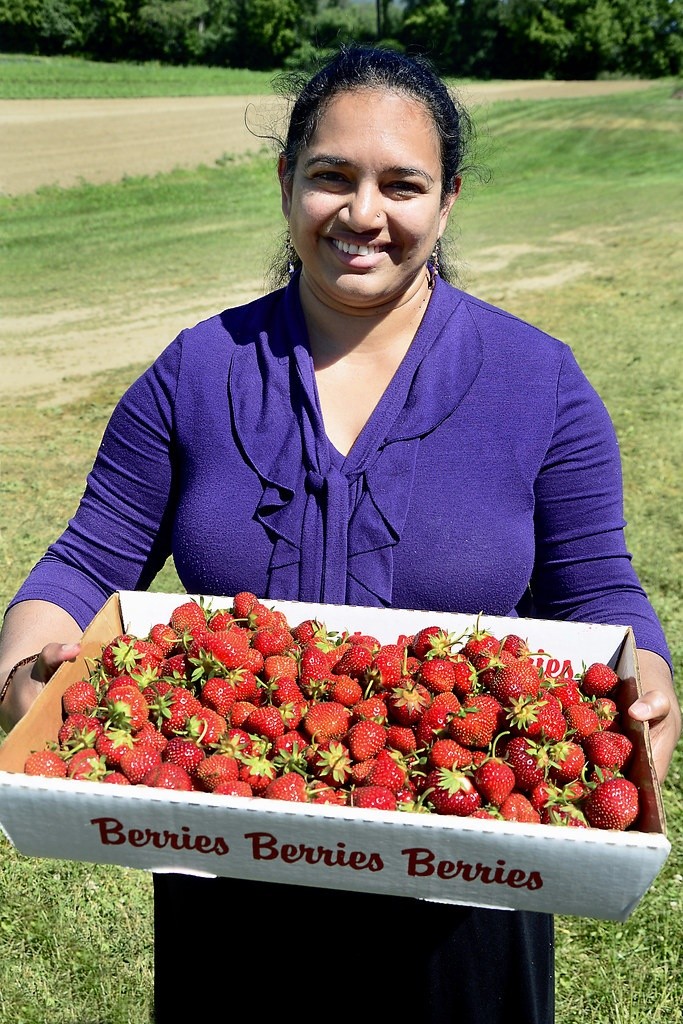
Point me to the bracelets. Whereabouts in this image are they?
[0,652,40,705]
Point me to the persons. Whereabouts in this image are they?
[0,45,683,1024]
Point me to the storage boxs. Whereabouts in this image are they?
[1,587,672,924]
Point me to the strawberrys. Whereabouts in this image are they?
[24,591,641,831]
